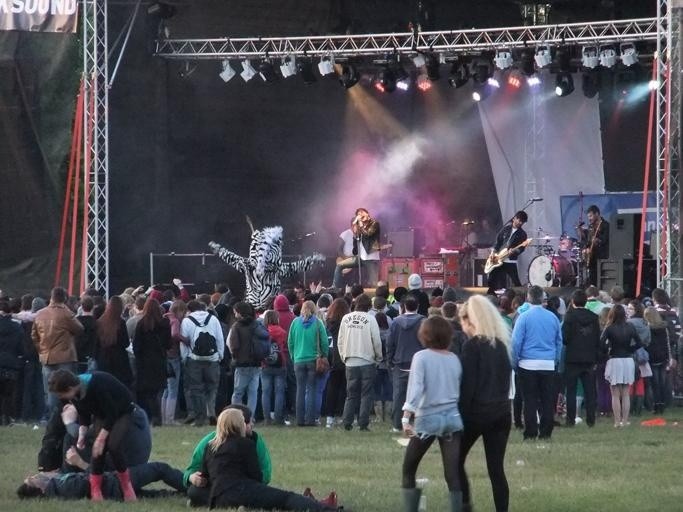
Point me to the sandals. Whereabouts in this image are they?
[615,421,631,429]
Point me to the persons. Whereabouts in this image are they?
[326,209,381,293]
[336,217,361,289]
[400,314,465,512]
[435,217,495,288]
[45,369,137,505]
[455,291,514,512]
[0,280,683,475]
[575,205,611,287]
[13,459,193,496]
[203,408,357,512]
[485,210,529,295]
[181,402,273,510]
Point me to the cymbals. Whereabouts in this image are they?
[532,237,560,240]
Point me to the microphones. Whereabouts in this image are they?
[463,221,476,225]
[353,215,362,225]
[575,222,585,229]
[531,197,543,202]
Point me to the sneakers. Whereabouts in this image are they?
[344,422,370,432]
[184,411,217,427]
[0,414,50,427]
[256,412,334,429]
[389,428,402,433]
[302,487,337,512]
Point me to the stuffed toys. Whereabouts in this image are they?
[206,224,327,311]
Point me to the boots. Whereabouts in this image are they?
[89,473,103,501]
[116,469,138,502]
[370,401,393,424]
[449,491,463,512]
[653,400,664,415]
[513,398,524,429]
[401,487,422,512]
[147,397,182,427]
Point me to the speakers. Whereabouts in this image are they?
[609,214,642,260]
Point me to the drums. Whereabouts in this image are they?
[527,253,574,290]
[571,247,580,262]
[557,235,576,252]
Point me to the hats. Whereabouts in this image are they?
[443,287,456,302]
[408,274,422,290]
[31,296,47,313]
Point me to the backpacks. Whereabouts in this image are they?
[247,320,269,361]
[264,340,282,368]
[183,314,219,362]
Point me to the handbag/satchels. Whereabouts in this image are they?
[166,362,176,378]
[316,357,330,376]
[666,358,678,371]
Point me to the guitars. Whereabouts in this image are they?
[336,243,393,275]
[483,238,531,273]
[581,219,601,266]
